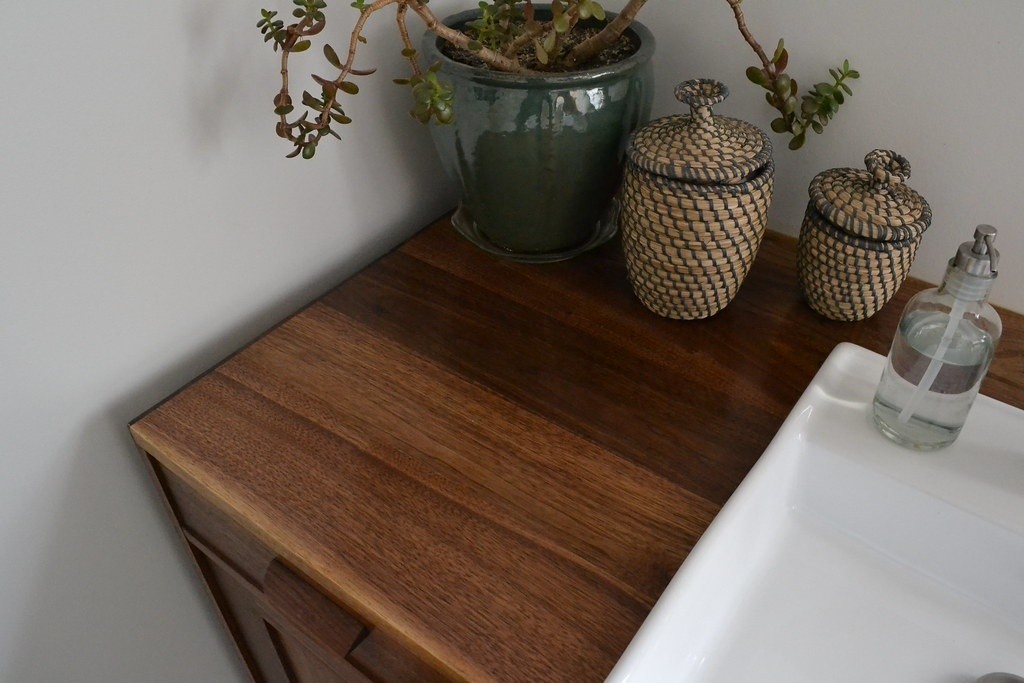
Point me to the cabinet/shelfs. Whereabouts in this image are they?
[128,207,1024,683]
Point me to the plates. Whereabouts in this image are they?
[603,343,1024,683]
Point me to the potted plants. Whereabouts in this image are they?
[256,0,860,256]
